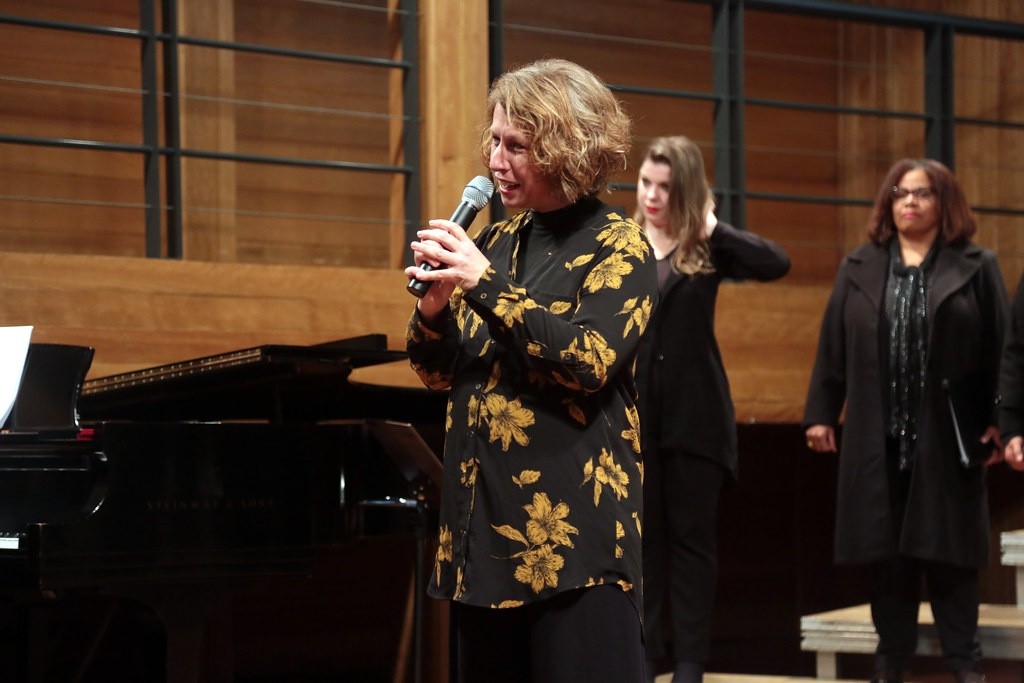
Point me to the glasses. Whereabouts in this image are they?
[888,186,935,199]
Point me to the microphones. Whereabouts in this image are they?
[406,176,495,300]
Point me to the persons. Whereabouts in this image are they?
[803,158,1010,683]
[405,59,661,683]
[632,136,790,683]
[996,265,1024,472]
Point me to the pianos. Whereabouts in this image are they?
[0,332,452,683]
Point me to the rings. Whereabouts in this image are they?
[807,441,814,448]
